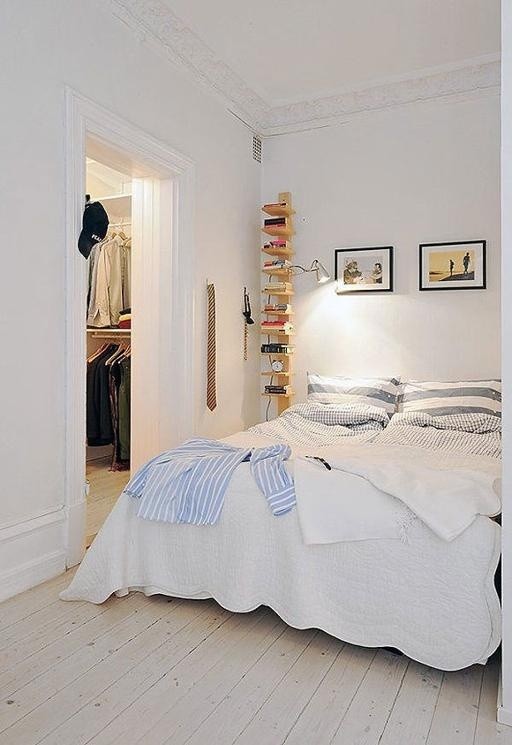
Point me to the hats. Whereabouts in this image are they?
[78,202,109,259]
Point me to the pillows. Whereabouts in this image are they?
[306,371,401,414]
[401,382,502,422]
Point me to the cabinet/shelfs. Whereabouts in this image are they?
[262,192,294,415]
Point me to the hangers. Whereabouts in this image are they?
[87,334,130,367]
[93,218,132,247]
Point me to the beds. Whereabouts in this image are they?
[62,364,503,678]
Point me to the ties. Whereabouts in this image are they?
[206,284,216,411]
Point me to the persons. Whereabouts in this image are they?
[463,252,470,276]
[370,262,383,283]
[344,258,366,284]
[449,259,455,277]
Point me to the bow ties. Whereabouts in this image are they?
[243,311,254,324]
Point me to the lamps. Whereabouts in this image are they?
[289,261,330,283]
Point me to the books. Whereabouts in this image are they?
[260,199,297,395]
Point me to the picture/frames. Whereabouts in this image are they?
[417,239,486,291]
[334,245,393,294]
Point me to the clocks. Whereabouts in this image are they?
[270,360,284,372]
